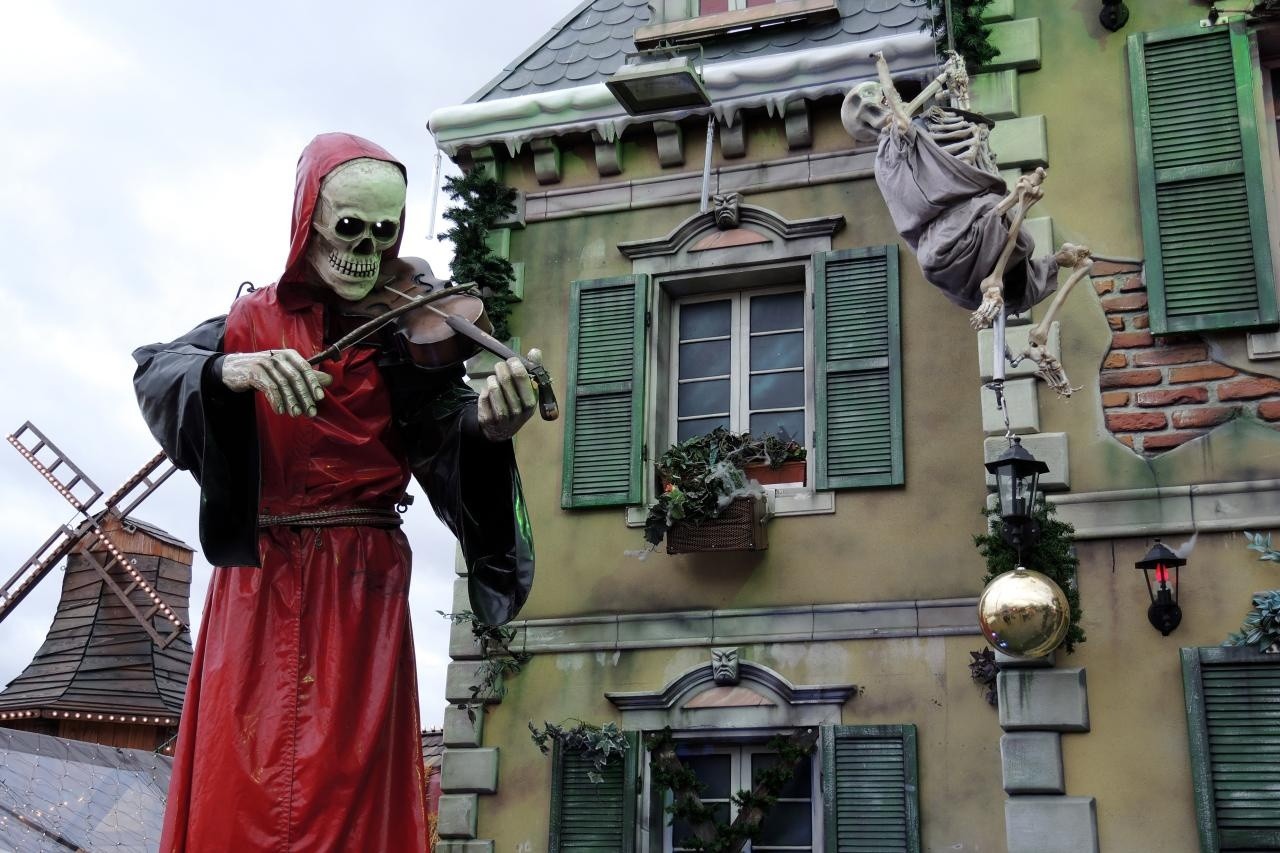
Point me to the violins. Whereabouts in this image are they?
[333,255,562,424]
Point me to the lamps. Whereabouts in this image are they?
[1135,539,1187,636]
[606,42,712,116]
[983,436,1051,552]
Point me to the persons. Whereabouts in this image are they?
[841,51,1092,395]
[133,133,543,853]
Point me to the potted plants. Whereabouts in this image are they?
[643,424,807,546]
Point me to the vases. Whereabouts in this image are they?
[666,495,776,555]
[745,460,806,485]
